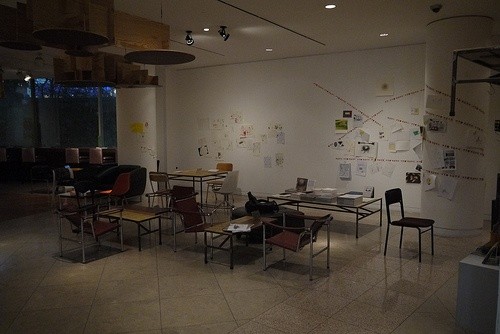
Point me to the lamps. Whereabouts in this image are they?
[217,25,231,42]
[185,30,195,46]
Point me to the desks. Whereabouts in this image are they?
[159,169,228,212]
[95,208,162,252]
[57,189,92,208]
[29,147,66,168]
[70,167,85,172]
[203,215,277,273]
[453,253,500,334]
[266,191,382,240]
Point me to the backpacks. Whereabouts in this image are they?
[245,192,280,214]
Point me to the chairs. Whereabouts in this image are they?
[29,159,333,284]
[384,187,435,263]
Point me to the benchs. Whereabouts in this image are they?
[230,205,306,246]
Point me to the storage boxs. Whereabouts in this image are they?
[65,148,80,164]
[0,148,6,162]
[337,191,364,207]
[88,147,103,165]
[291,192,306,200]
[21,146,36,163]
